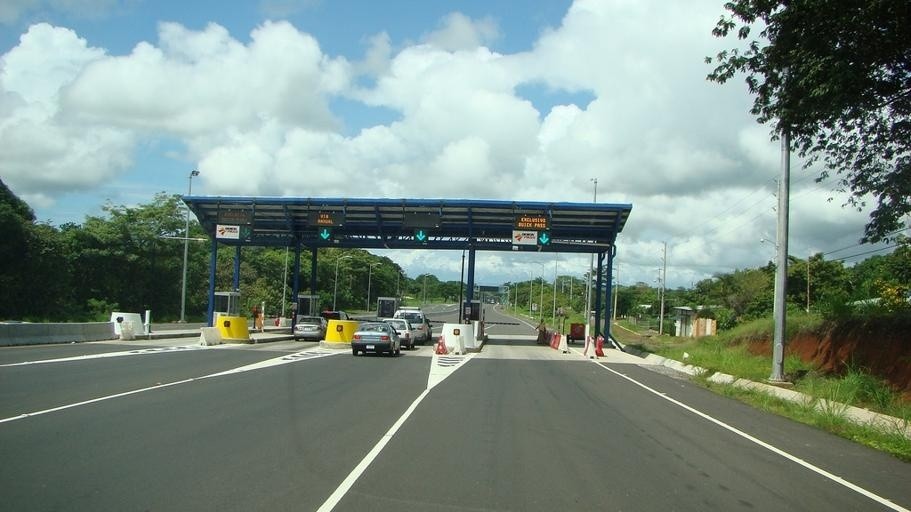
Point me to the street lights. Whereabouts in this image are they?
[333,255,353,312]
[177,169,200,323]
[498,260,544,323]
[397,269,406,296]
[367,262,383,313]
[423,271,432,305]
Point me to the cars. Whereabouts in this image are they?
[351,309,433,357]
[294,311,349,341]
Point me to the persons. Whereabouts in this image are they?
[535,318,548,345]
